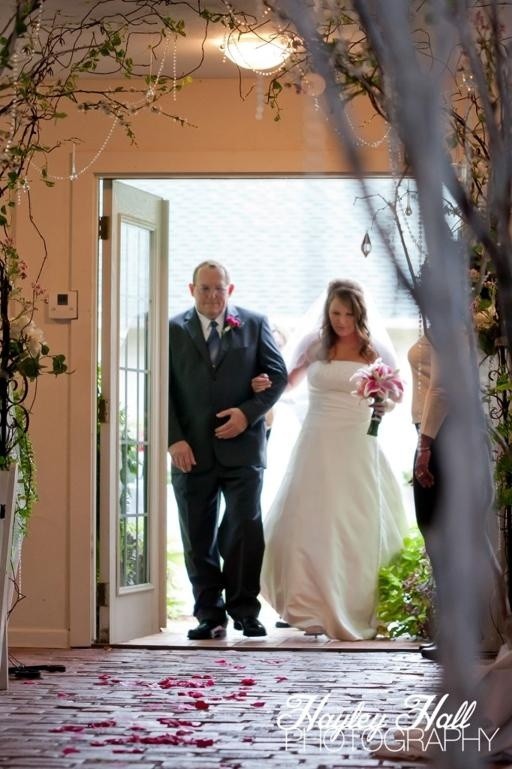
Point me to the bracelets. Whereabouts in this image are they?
[416,446,430,452]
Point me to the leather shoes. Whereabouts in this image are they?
[275,616,290,628]
[233,616,266,636]
[187,620,225,639]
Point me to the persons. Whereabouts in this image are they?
[407,260,477,665]
[168,260,287,640]
[252,278,414,640]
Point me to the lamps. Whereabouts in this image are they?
[221,38,295,69]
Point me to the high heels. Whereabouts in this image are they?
[303,623,324,639]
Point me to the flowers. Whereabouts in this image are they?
[351,358,406,436]
[223,316,243,335]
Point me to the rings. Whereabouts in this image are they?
[417,472,426,480]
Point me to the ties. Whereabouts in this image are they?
[206,320,222,367]
